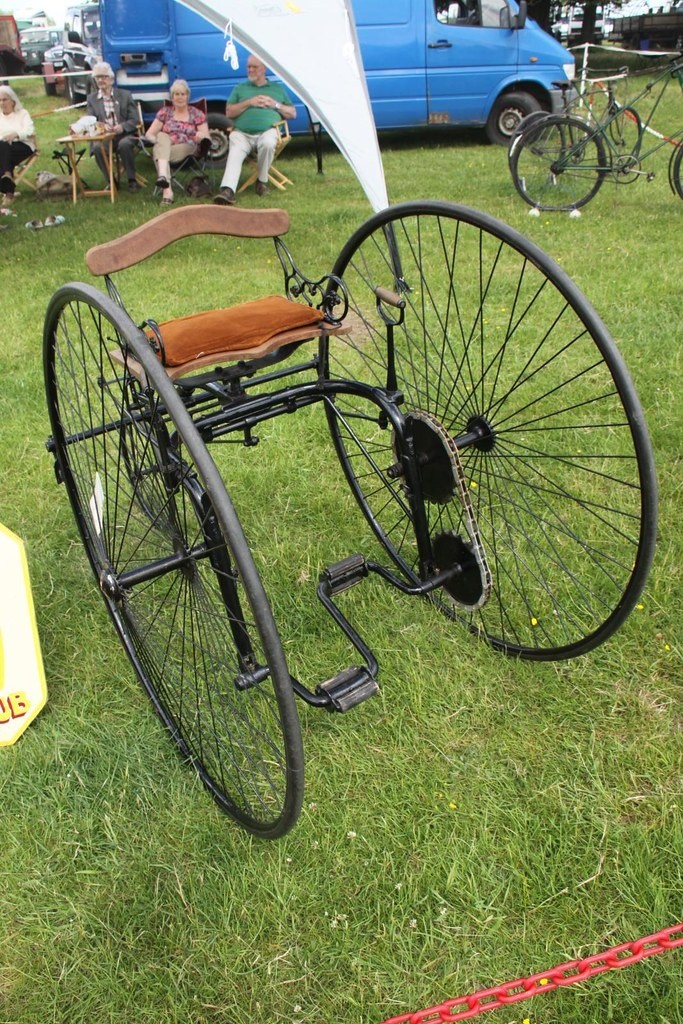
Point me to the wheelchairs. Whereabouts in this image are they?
[43,199,655,842]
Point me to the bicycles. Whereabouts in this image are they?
[509,49,683,212]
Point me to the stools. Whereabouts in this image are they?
[0,148,41,200]
[51,147,89,188]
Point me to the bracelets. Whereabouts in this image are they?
[275,102,280,110]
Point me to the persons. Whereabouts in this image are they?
[0,85,36,205]
[214,55,296,205]
[145,80,211,207]
[86,62,142,192]
[553,27,562,43]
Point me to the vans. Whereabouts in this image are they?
[18,27,66,73]
[100,0,577,171]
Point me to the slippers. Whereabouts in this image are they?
[45,215,65,226]
[25,219,43,228]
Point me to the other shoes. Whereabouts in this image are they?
[128,182,139,194]
[2,197,15,205]
[160,198,175,206]
[255,178,267,195]
[213,188,236,205]
[155,176,169,188]
[104,183,121,191]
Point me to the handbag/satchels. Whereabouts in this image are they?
[185,176,212,199]
[35,171,80,202]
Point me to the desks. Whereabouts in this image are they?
[55,132,117,208]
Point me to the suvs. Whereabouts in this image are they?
[63,4,103,102]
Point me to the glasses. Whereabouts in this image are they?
[94,74,109,79]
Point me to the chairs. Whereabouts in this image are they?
[226,119,295,193]
[152,98,212,197]
[103,101,150,192]
[83,203,354,388]
